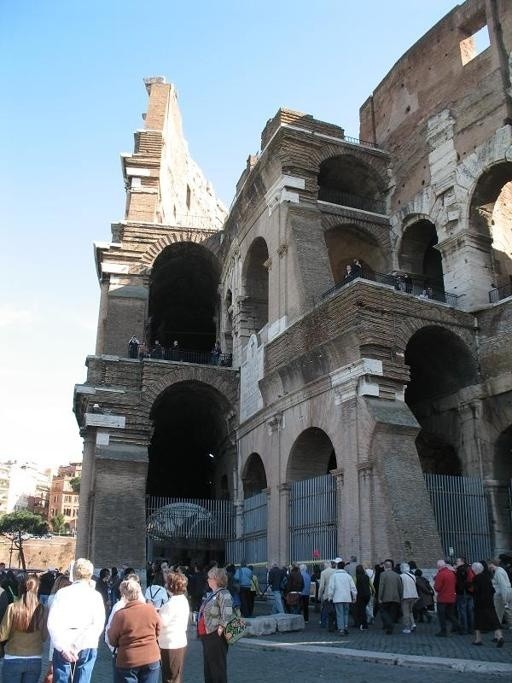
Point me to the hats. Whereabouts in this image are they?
[330,557,343,563]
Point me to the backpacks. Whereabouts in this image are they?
[146,584,161,605]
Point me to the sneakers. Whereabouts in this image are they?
[451,626,474,635]
[359,625,369,630]
[436,631,448,637]
[382,612,433,635]
[305,616,311,623]
[472,640,483,646]
[318,619,349,636]
[491,637,504,648]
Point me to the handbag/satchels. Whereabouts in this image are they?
[287,592,301,605]
[225,617,248,646]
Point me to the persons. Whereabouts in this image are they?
[211,342,222,364]
[128,334,181,361]
[147,560,263,618]
[344,258,434,300]
[105,573,190,683]
[95,564,136,607]
[221,352,232,367]
[0,562,72,683]
[268,563,311,623]
[311,558,435,635]
[433,554,512,647]
[47,558,106,683]
[197,567,233,683]
